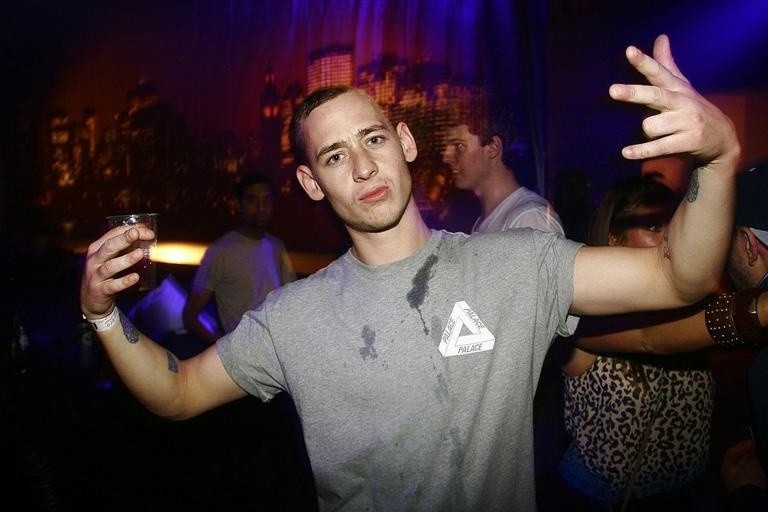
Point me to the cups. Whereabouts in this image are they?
[104,213,160,294]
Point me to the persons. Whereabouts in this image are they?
[434,92,568,237]
[79,33,742,512]
[717,435,768,511]
[183,172,297,345]
[546,175,767,498]
[718,161,768,293]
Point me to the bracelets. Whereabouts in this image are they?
[88,305,121,333]
[731,287,768,350]
[701,288,743,350]
